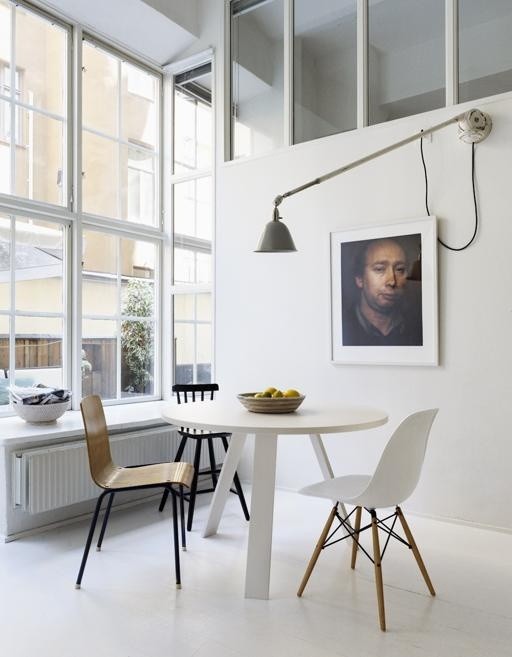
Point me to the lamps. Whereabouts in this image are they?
[255,107,491,255]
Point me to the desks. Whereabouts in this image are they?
[161,399,388,600]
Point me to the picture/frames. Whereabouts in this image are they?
[328,216,440,367]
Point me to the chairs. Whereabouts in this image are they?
[157,383,250,531]
[296,407,441,632]
[74,393,195,590]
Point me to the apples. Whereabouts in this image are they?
[255,388,300,398]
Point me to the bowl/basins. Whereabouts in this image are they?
[12,398,71,426]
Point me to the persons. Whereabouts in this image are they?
[341,240,418,346]
